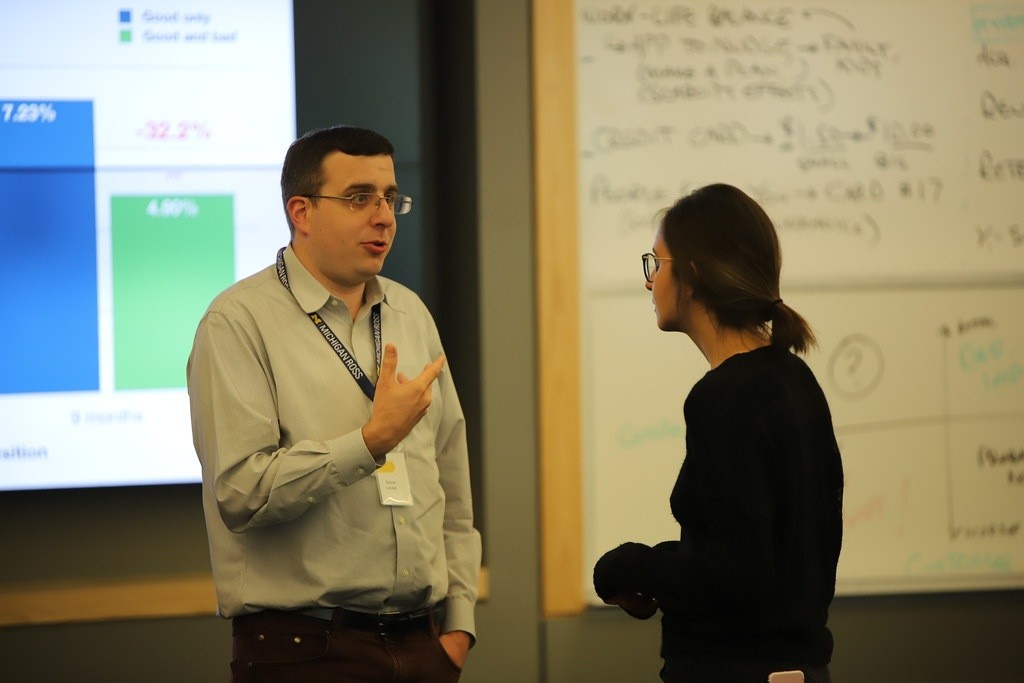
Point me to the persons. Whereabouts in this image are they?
[593,184,844,683]
[187,126,482,682]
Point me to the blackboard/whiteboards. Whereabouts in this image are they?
[527,1,1024,624]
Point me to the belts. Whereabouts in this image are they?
[293,605,440,634]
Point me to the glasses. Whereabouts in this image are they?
[642,252,672,281]
[298,192,412,214]
